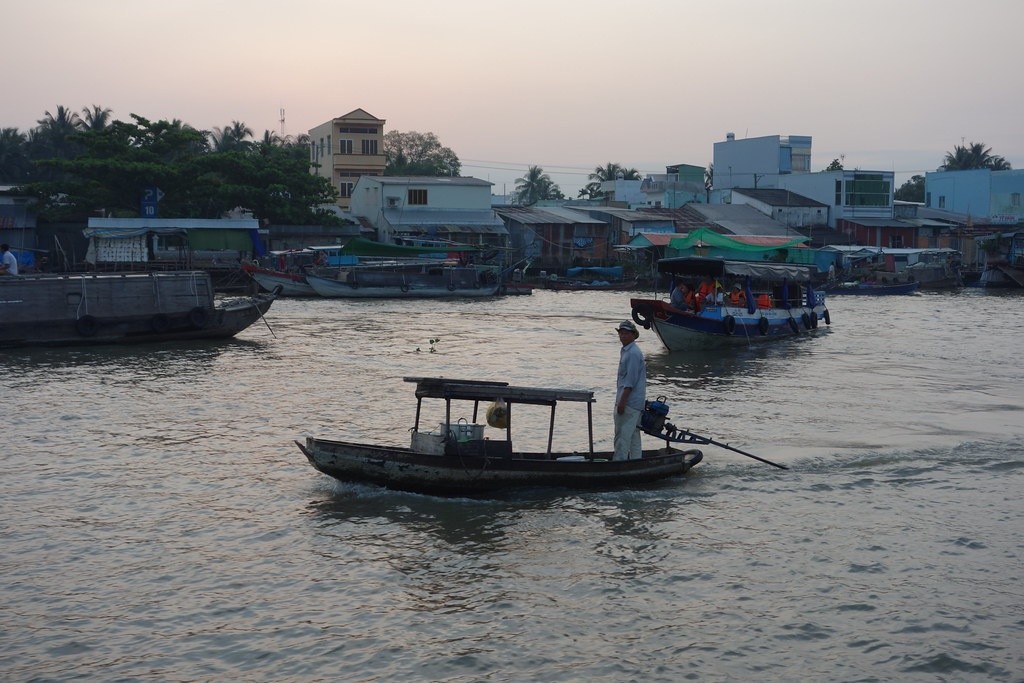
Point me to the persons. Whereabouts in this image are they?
[829,261,835,286]
[0,244,18,276]
[671,281,745,312]
[613,320,646,461]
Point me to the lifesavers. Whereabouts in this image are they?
[759,317,769,335]
[789,317,800,334]
[401,284,409,292]
[723,315,736,335]
[810,312,818,329]
[824,310,830,324]
[631,302,653,329]
[447,283,456,291]
[871,275,915,283]
[188,307,209,329]
[351,281,360,289]
[76,315,99,337]
[802,313,811,330]
[497,285,507,296]
[151,313,170,333]
[473,281,481,289]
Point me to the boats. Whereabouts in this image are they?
[630,254,830,353]
[543,266,639,290]
[237,232,536,297]
[294,376,704,503]
[821,246,964,294]
[0,267,283,349]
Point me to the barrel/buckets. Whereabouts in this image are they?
[440,417,486,441]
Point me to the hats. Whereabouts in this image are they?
[615,320,639,340]
[732,283,741,290]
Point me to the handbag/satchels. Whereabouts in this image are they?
[486,398,507,428]
